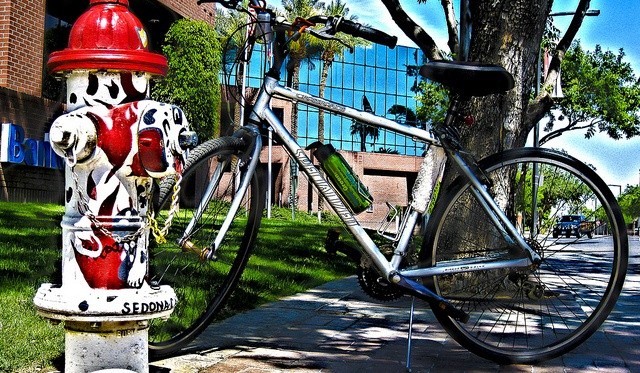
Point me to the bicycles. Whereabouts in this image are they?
[148,1,628,370]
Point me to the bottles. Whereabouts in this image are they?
[305,140,374,215]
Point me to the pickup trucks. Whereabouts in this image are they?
[553,214,597,239]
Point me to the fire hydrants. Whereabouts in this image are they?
[34,0,199,372]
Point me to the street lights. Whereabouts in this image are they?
[529,6,601,242]
